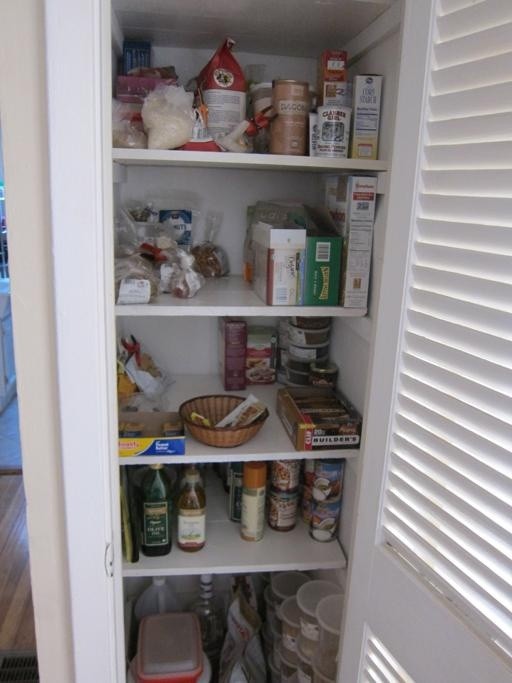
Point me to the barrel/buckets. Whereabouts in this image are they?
[189,576,227,682]
[134,576,185,653]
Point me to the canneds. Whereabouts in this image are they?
[268,458,345,543]
[268,80,309,156]
[315,106,352,158]
[310,362,338,389]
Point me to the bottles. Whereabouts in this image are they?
[139,461,172,557]
[189,573,224,663]
[213,461,267,542]
[176,470,206,551]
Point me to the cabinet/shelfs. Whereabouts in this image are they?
[95,2,407,683]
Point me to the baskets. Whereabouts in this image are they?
[178,393,270,449]
